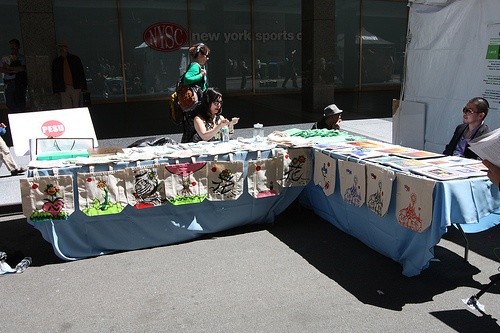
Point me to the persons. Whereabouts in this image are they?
[311,103,344,130]
[90,43,405,96]
[442,96,491,160]
[181,42,211,142]
[482,157,500,191]
[0,121,27,175]
[192,86,240,142]
[50,40,88,109]
[0,37,27,112]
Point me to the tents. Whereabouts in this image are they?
[336,26,396,81]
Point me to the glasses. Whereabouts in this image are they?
[204,53,209,58]
[463,107,471,113]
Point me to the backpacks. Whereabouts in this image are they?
[176,62,204,113]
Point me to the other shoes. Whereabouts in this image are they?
[11,168,27,175]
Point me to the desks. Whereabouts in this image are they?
[25,129,497,277]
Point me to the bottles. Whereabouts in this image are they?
[253,122,264,142]
[222,126,230,143]
[16,256,33,274]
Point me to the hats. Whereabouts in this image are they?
[323,103,343,117]
